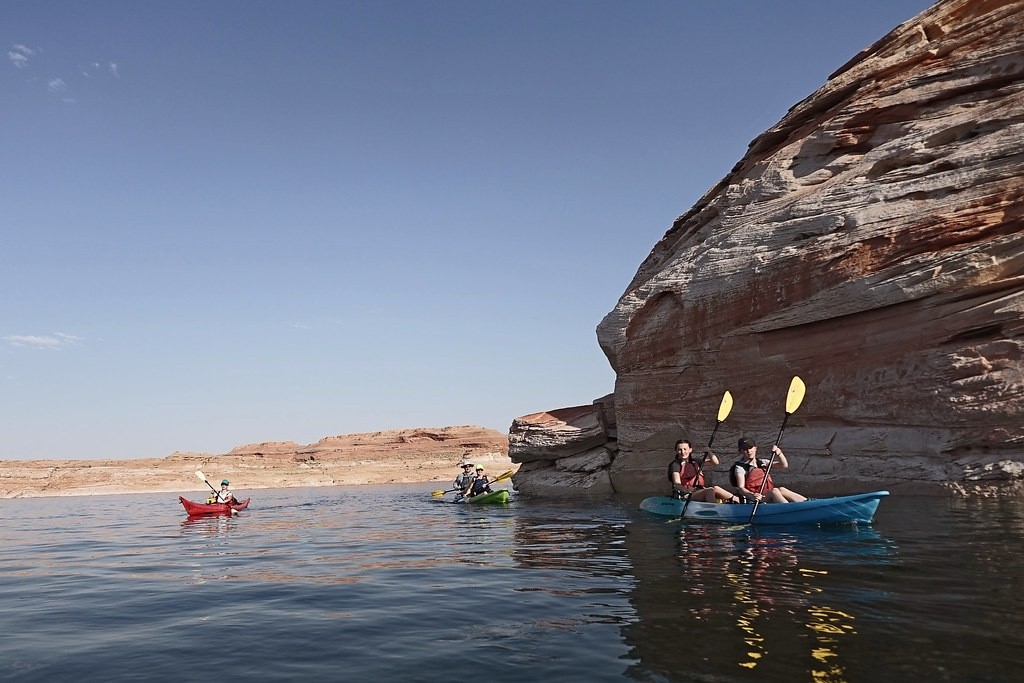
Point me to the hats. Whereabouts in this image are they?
[476,463,484,471]
[461,460,474,468]
[221,479,229,485]
[738,437,756,452]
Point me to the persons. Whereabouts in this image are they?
[667,439,740,504]
[729,437,810,504]
[210,479,233,503]
[453,460,477,498]
[463,464,492,498]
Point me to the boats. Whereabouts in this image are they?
[639,491,889,527]
[179,496,250,517]
[454,488,510,504]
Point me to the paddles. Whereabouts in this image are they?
[747,375,807,526]
[452,469,515,504]
[430,476,509,497]
[195,470,238,513]
[680,390,734,518]
